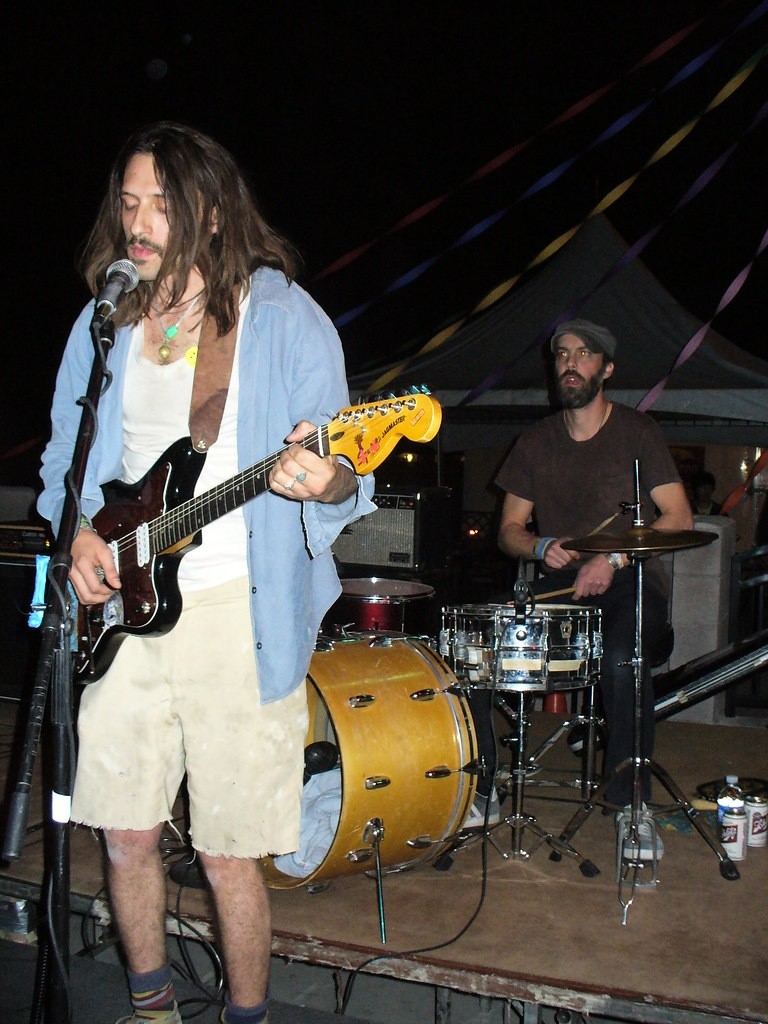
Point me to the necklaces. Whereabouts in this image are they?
[154,294,200,365]
[599,401,607,428]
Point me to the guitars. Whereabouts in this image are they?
[55,381,447,686]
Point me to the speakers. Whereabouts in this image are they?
[0,554,43,703]
[329,487,433,580]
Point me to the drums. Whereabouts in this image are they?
[438,603,603,693]
[322,575,438,642]
[257,628,481,893]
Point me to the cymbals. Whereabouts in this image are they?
[558,526,721,552]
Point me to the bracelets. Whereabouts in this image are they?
[604,553,624,572]
[532,537,557,560]
[80,517,91,528]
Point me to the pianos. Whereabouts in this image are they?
[0,518,71,865]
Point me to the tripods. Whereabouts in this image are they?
[431,550,740,925]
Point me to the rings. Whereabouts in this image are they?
[284,481,295,490]
[296,470,306,481]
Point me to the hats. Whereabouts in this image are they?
[551,319,617,361]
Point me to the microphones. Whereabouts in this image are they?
[304,740,341,775]
[514,562,529,592]
[91,259,140,330]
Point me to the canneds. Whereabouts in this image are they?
[720,809,748,860]
[744,796,768,847]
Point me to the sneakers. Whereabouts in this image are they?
[463,790,499,828]
[615,802,665,861]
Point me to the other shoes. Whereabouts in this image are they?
[220,1006,269,1024]
[115,999,183,1024]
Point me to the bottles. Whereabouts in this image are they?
[718,776,746,839]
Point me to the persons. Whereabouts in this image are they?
[448,319,695,860]
[689,471,730,518]
[34,119,377,1024]
